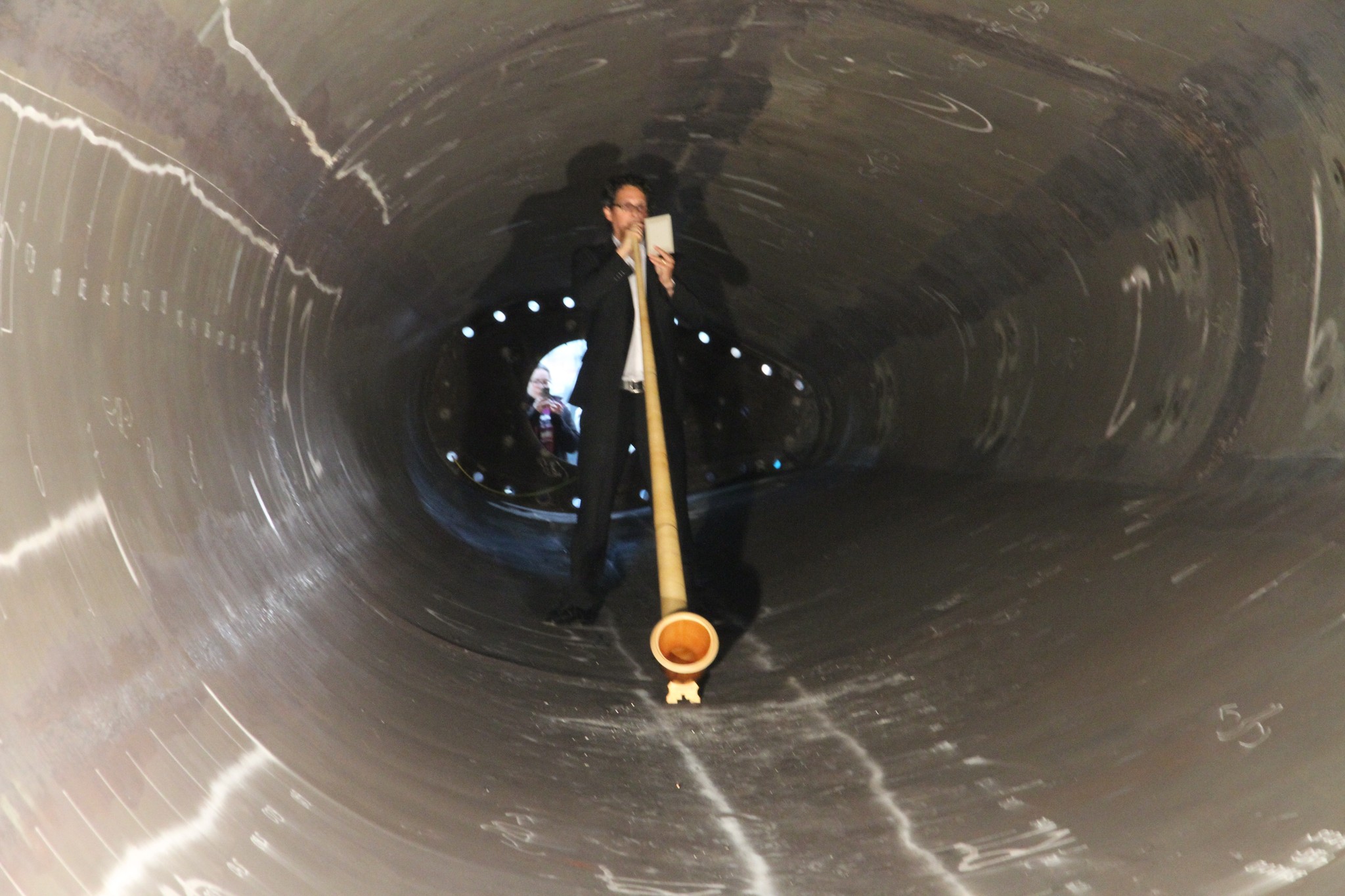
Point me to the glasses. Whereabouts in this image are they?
[611,202,649,214]
[531,379,550,387]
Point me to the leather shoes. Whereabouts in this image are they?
[542,607,601,628]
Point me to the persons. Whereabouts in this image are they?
[525,362,580,461]
[549,175,701,625]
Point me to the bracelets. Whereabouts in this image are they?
[664,279,677,288]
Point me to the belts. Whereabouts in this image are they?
[618,380,644,394]
